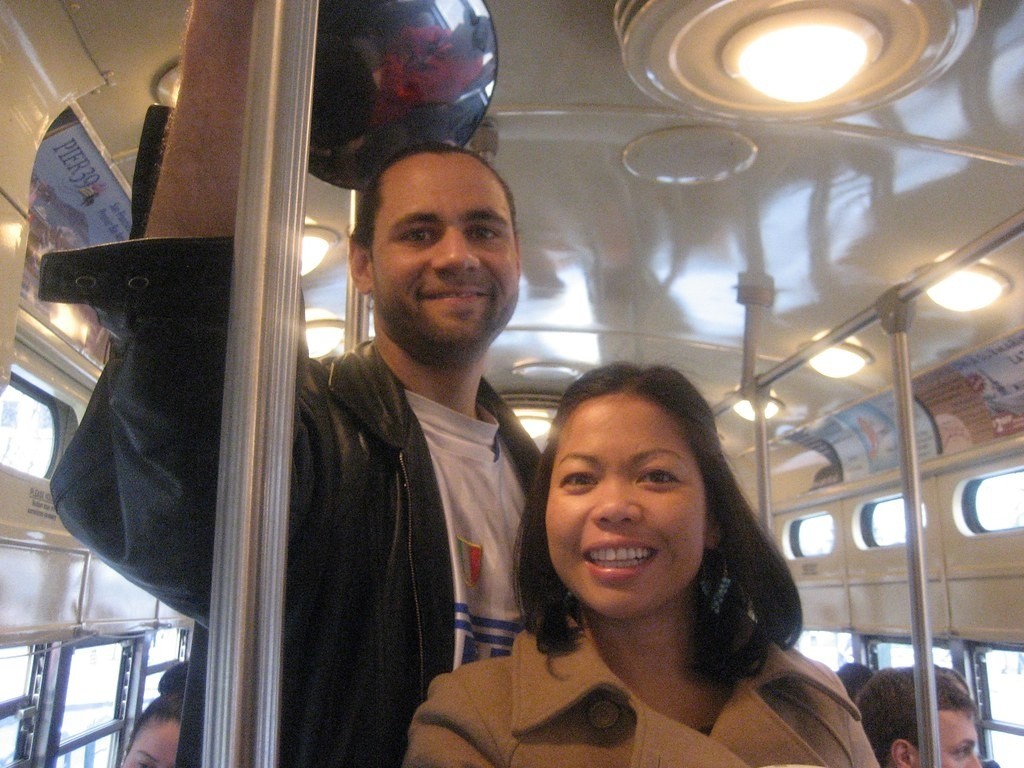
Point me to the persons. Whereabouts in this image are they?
[837,662,1004,768]
[34,0,544,768]
[120,661,188,768]
[402,359,880,768]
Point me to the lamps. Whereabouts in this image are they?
[614,0,986,124]
